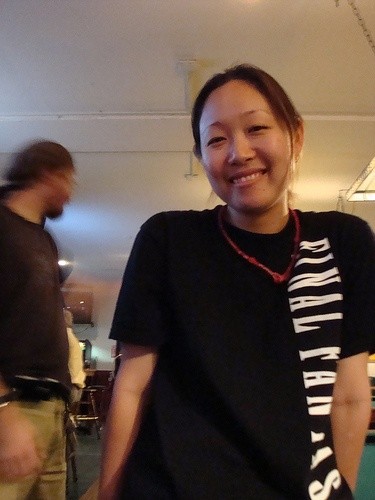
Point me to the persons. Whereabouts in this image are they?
[0,140,86,500]
[97,63,375,500]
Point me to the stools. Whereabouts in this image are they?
[81,385,104,439]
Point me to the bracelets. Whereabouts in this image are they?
[0,392,21,404]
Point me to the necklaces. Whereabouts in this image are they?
[218,204,301,282]
